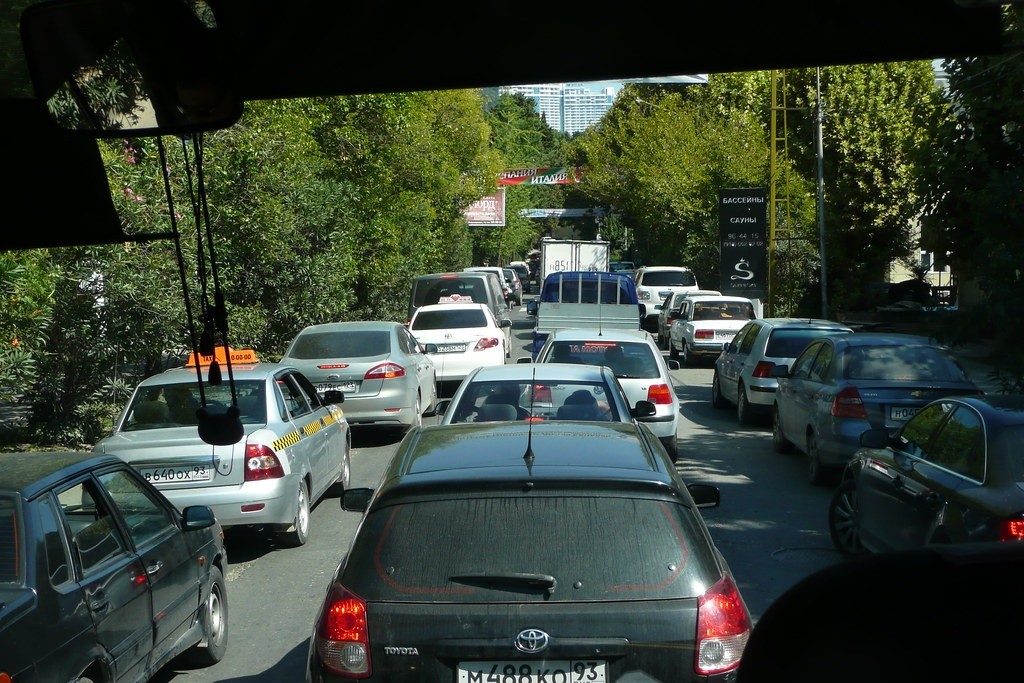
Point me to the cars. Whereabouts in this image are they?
[301,365,757,683]
[273,321,439,435]
[434,361,656,430]
[464,260,536,310]
[653,289,724,349]
[0,449,233,683]
[668,296,761,366]
[608,260,638,281]
[405,294,512,393]
[767,332,987,487]
[516,300,683,464]
[828,392,1023,563]
[711,311,854,425]
[81,346,351,547]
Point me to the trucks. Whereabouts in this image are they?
[539,237,611,300]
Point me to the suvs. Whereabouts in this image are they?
[633,265,700,327]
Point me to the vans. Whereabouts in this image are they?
[409,270,512,364]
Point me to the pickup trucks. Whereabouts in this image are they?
[525,271,647,363]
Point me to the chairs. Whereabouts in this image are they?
[172,398,204,423]
[134,399,183,429]
[555,404,600,422]
[611,355,644,377]
[230,395,264,422]
[552,354,584,367]
[479,402,520,421]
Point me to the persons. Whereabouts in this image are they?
[493,383,528,419]
[560,390,605,421]
[552,342,575,363]
[602,344,635,375]
